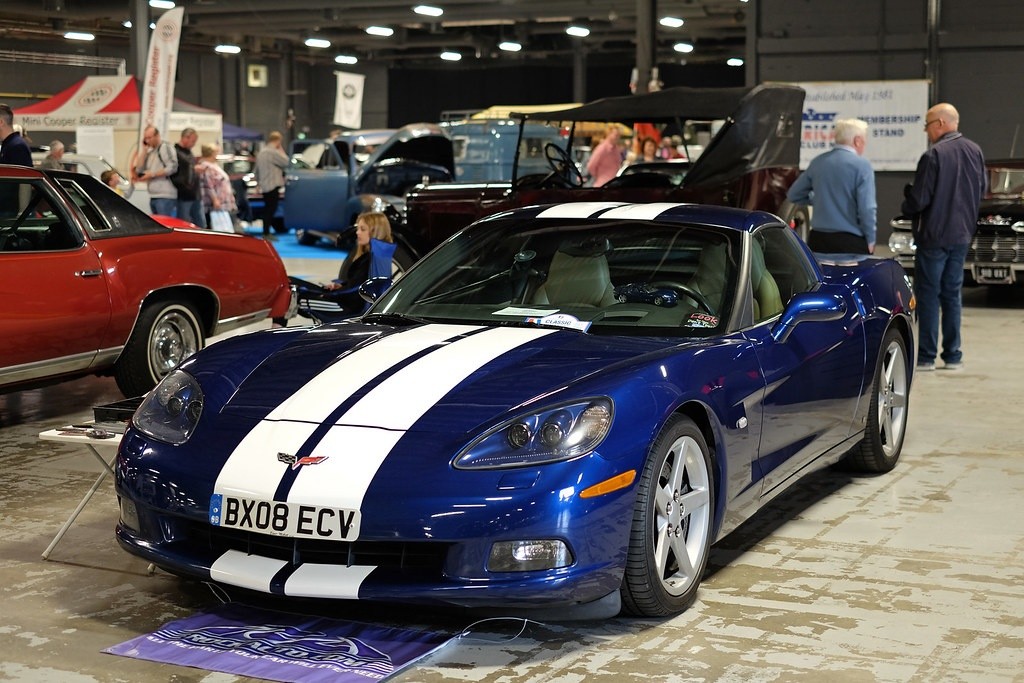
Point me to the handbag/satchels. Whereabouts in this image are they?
[210,210,236,233]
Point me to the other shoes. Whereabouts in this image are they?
[917,362,935,370]
[945,361,964,368]
[264,235,279,241]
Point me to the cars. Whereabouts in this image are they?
[281,128,459,245]
[406,81,811,261]
[115,198,921,622]
[0,162,300,398]
[888,155,1024,287]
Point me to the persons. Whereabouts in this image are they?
[270,213,391,328]
[0,105,685,234]
[787,117,877,255]
[900,103,984,371]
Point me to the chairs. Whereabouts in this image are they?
[531,238,618,308]
[289,238,399,327]
[684,233,783,321]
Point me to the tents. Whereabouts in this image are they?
[12,74,224,173]
[222,119,263,154]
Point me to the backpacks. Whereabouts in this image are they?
[157,141,196,190]
